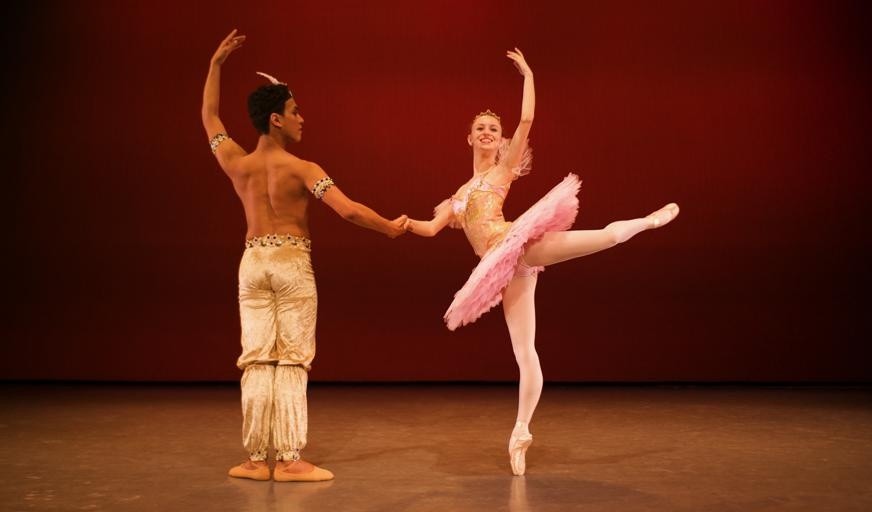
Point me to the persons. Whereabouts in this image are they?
[399,47,679,476]
[201,27,412,481]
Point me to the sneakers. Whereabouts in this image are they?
[274,467,334,482]
[228,464,270,480]
[508,432,533,475]
[648,203,680,229]
